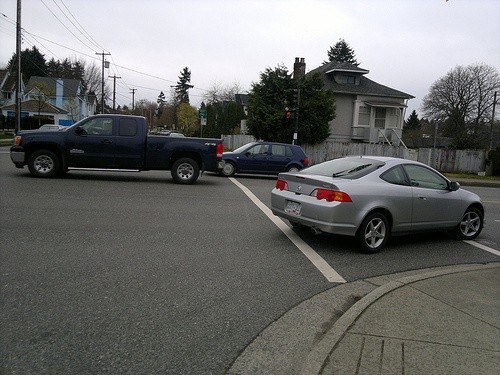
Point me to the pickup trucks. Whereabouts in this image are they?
[10,113,224,184]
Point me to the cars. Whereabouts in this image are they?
[39,124,63,129]
[270,155,485,252]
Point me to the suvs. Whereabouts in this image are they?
[220,142,308,178]
